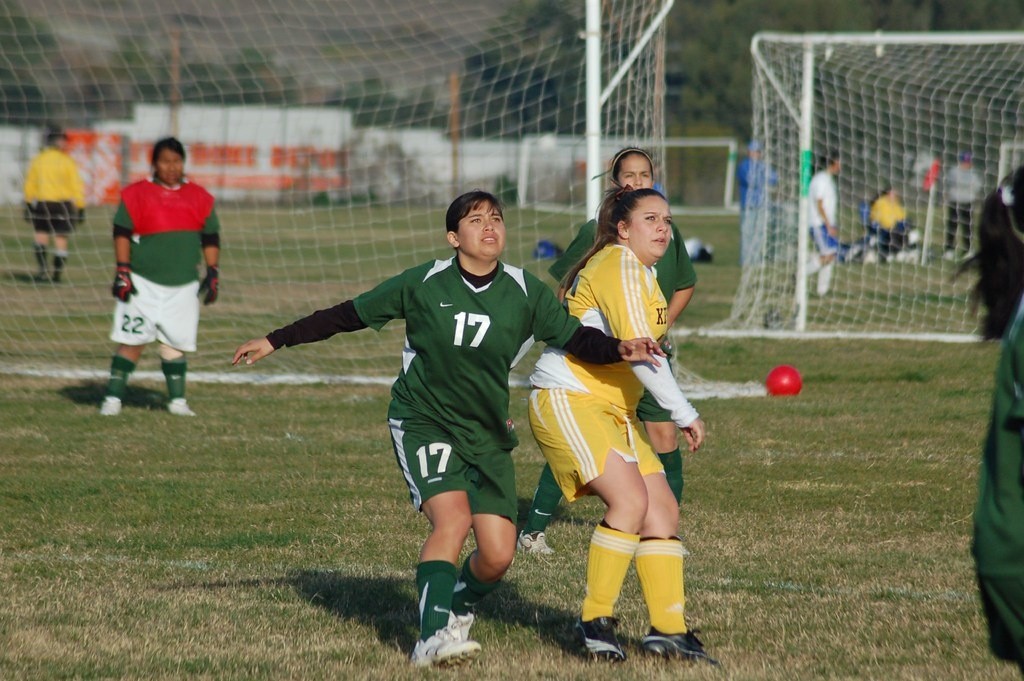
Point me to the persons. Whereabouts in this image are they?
[24,125,87,283]
[525,186,725,672]
[99,137,220,420]
[736,137,982,297]
[228,190,668,670]
[514,142,698,559]
[947,159,1024,664]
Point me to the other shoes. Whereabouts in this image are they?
[169,398,196,417]
[101,395,121,416]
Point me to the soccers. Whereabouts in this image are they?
[767,365,803,396]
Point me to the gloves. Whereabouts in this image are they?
[198,263,218,305]
[112,262,138,303]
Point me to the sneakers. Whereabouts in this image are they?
[642,627,718,665]
[515,529,554,555]
[447,610,474,641]
[576,614,626,661]
[409,628,482,671]
